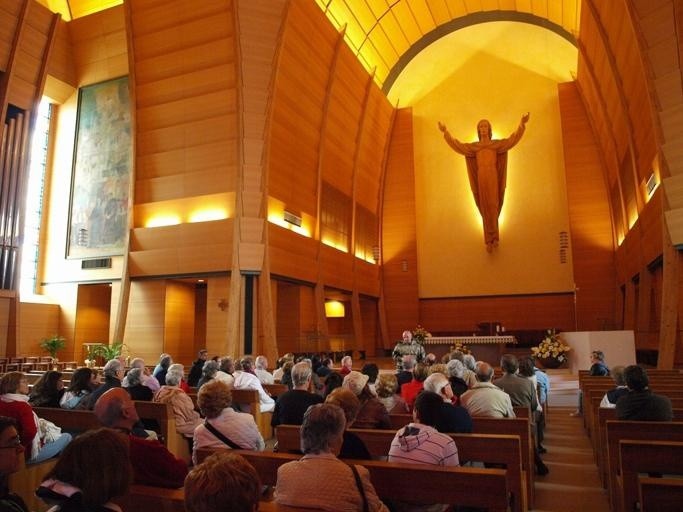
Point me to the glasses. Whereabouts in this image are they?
[0,435,20,448]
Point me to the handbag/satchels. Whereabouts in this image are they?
[39,418,61,442]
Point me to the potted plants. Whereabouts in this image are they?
[38,333,65,362]
[80,343,100,368]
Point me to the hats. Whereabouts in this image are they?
[342,371,369,396]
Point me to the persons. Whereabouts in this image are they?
[0,353,388,510]
[388,350,550,476]
[392,331,426,372]
[600,367,632,410]
[617,366,673,421]
[439,111,531,254]
[570,350,611,417]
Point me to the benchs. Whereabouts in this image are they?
[570,350,679,508]
[1,352,556,508]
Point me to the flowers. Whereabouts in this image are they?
[527,330,572,363]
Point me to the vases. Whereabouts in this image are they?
[535,352,567,369]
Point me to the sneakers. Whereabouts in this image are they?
[570,411,582,417]
[538,464,548,475]
